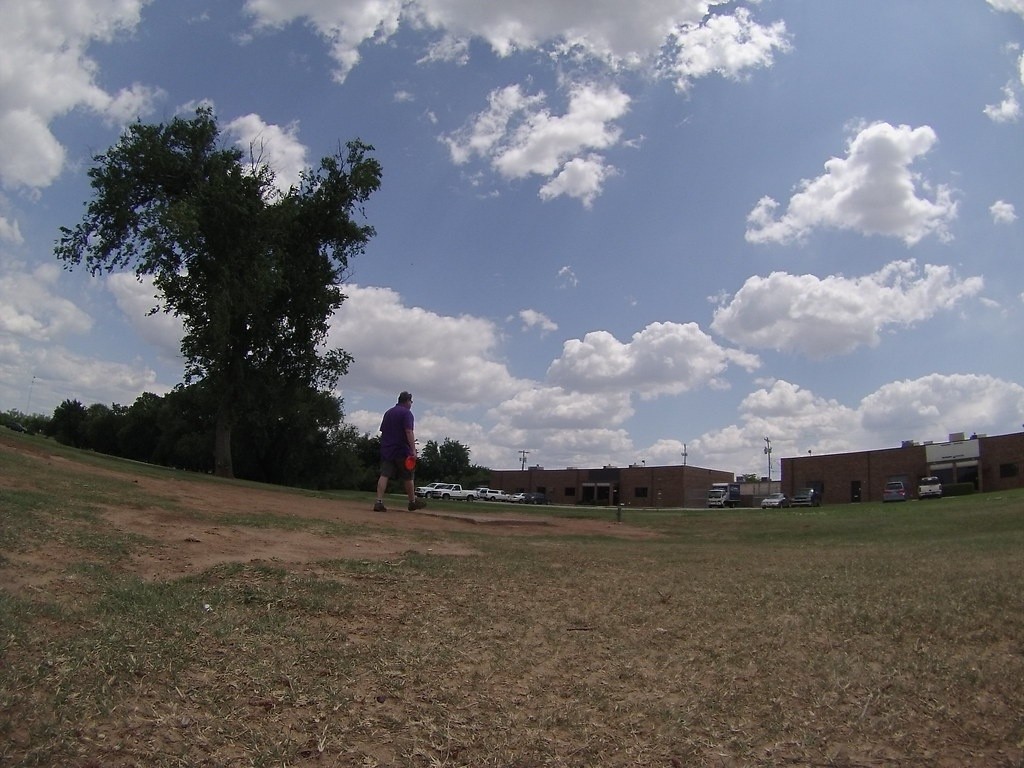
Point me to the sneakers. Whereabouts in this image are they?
[374,503,387,512]
[407,501,426,511]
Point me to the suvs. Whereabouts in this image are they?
[475,488,490,499]
[415,482,447,499]
[485,489,510,502]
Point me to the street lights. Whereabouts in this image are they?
[763,436,773,495]
[518,449,530,472]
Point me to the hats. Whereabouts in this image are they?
[399,392,412,399]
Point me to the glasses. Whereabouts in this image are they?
[411,401,413,403]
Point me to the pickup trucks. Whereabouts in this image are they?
[431,484,478,502]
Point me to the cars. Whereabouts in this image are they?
[761,493,791,509]
[883,481,907,502]
[509,493,535,504]
[918,477,943,498]
[790,488,822,508]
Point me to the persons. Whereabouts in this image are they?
[374,392,426,513]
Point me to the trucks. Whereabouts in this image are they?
[708,482,731,507]
